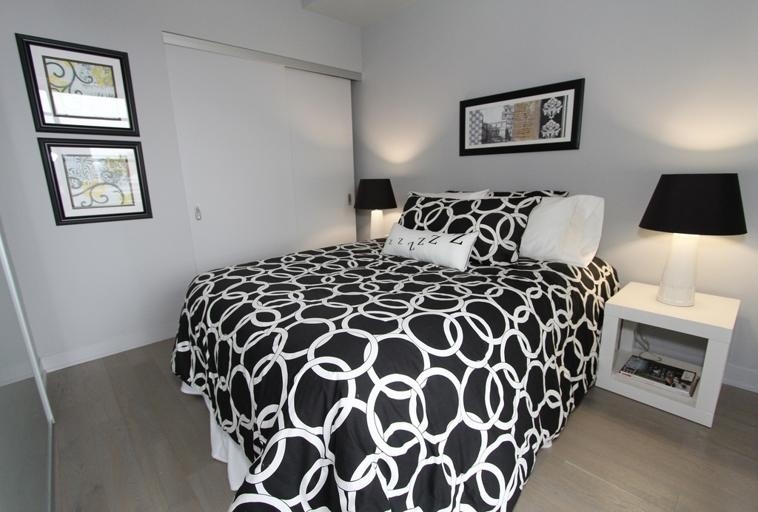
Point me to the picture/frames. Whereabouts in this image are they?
[456,77,585,158]
[13,31,154,227]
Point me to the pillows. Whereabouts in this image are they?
[378,185,604,273]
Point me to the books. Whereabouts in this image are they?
[619,355,700,399]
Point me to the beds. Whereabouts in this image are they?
[171,234,618,512]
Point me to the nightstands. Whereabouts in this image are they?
[595,280,740,430]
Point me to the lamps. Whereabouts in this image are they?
[638,172,746,307]
[352,178,399,240]
